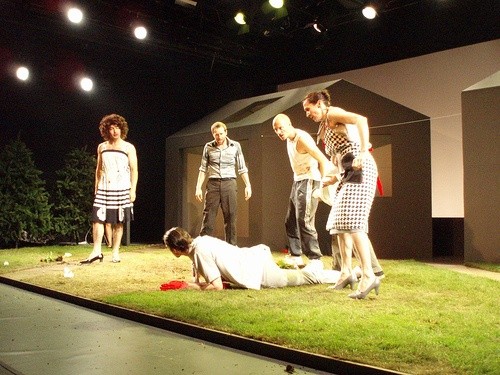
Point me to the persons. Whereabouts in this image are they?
[302,89,380,298]
[195,121,252,245]
[272,114,329,270]
[79,113,138,263]
[323,142,386,280]
[105,222,122,248]
[164,226,361,290]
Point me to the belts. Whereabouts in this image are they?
[209,177,236,181]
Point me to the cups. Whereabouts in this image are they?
[62,254,74,278]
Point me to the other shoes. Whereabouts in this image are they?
[355,271,386,281]
[302,259,323,270]
[283,254,305,266]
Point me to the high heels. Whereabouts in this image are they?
[348,278,379,300]
[112,251,121,263]
[80,253,104,264]
[327,273,354,291]
